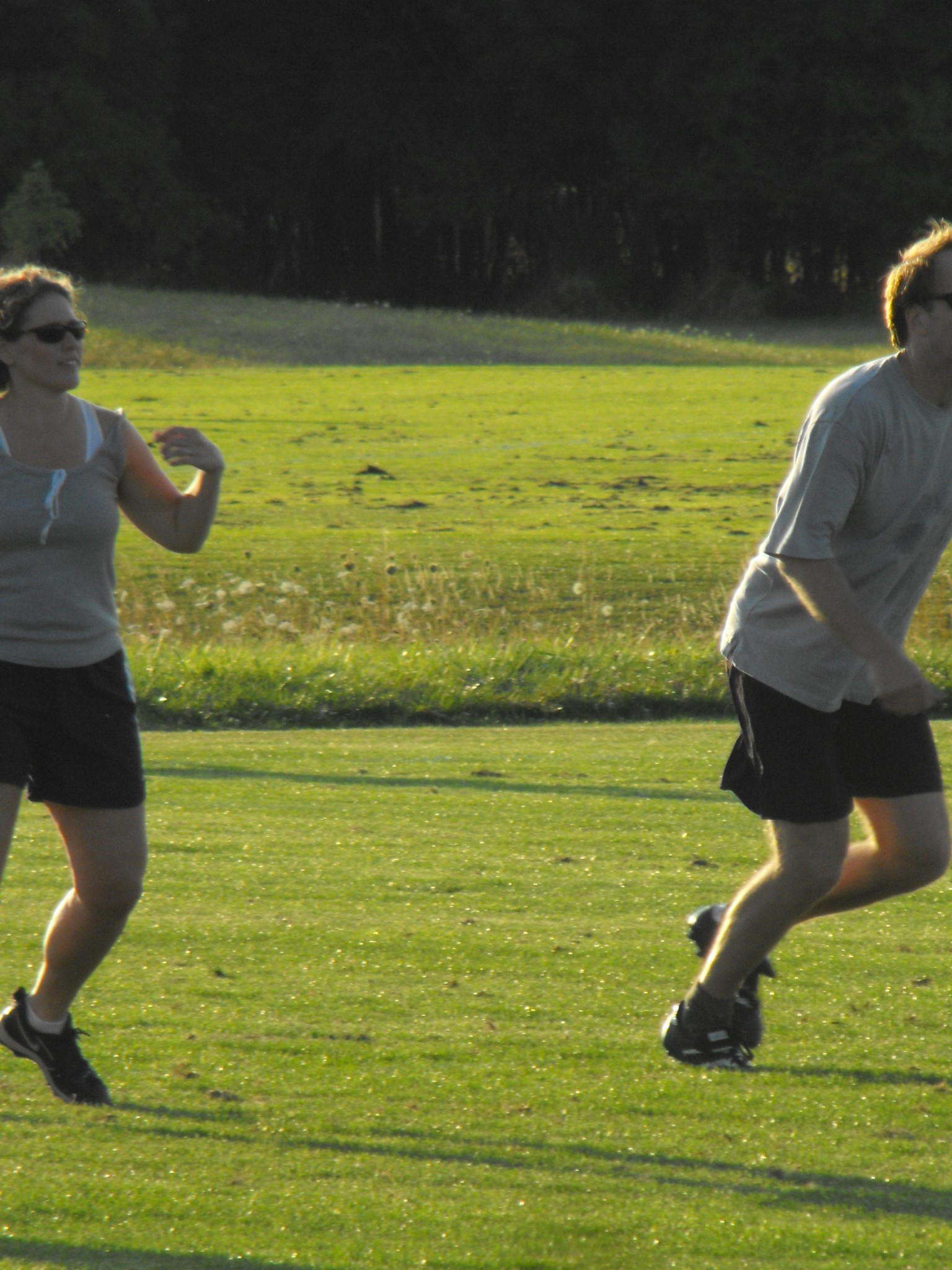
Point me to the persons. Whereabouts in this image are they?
[660,226,952,1073]
[0,264,226,1107]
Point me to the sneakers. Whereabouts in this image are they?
[687,906,778,1050]
[663,1000,757,1074]
[0,988,109,1106]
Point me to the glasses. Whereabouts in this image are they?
[0,320,91,345]
